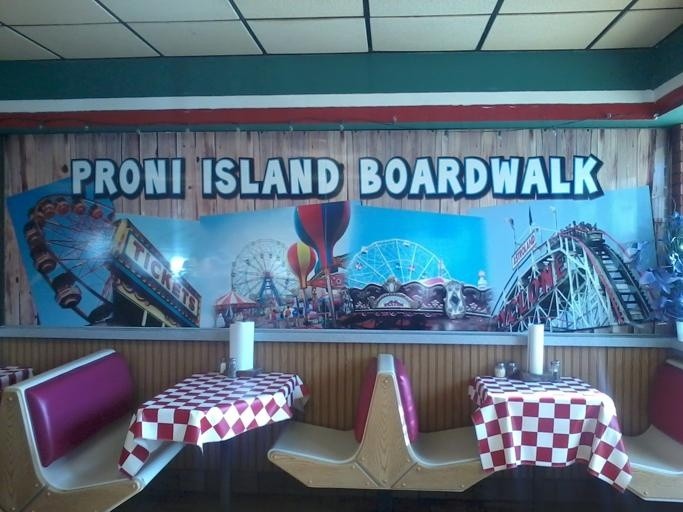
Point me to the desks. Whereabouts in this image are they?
[119,371,311,512]
[469,374,633,510]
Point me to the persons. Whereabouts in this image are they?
[231,297,330,328]
[548,219,599,237]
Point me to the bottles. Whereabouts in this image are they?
[550,360,561,380]
[227,358,237,377]
[506,361,518,377]
[219,357,226,374]
[493,362,505,377]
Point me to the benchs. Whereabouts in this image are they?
[0,347,183,512]
[615,359,683,512]
[267,351,494,511]
